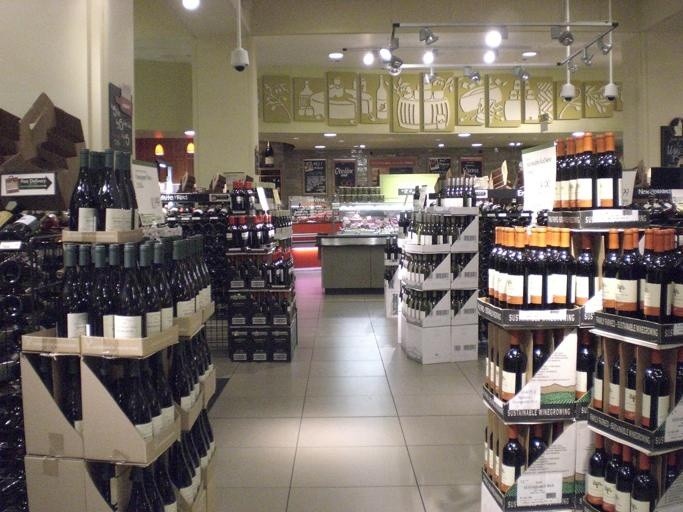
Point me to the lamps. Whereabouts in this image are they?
[153,0,623,158]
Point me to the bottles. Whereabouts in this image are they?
[65,144,142,231]
[552,131,622,211]
[0,203,215,512]
[263,139,274,168]
[475,197,681,512]
[383,212,475,322]
[231,179,295,320]
[412,177,475,208]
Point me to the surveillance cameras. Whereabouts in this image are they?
[603,83,619,101]
[230,47,249,72]
[559,83,577,102]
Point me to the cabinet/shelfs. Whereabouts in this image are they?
[162,187,298,363]
[477,188,682,512]
[0,187,218,512]
[384,204,549,364]
[316,202,415,294]
